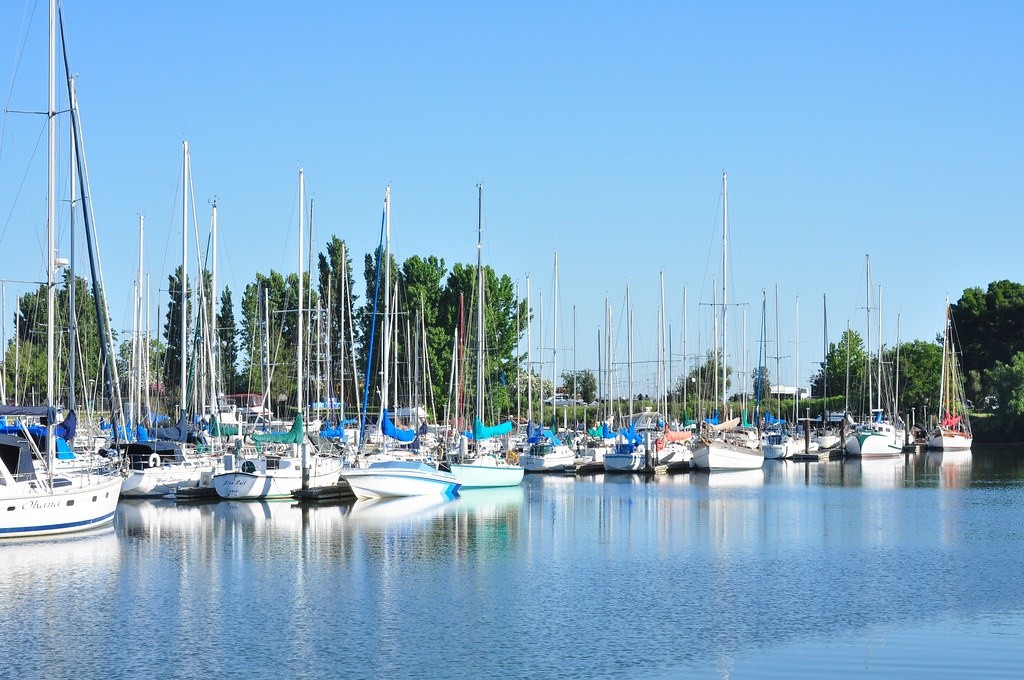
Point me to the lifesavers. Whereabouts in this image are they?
[147,452,160,468]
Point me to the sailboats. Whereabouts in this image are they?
[0,0,974,551]
